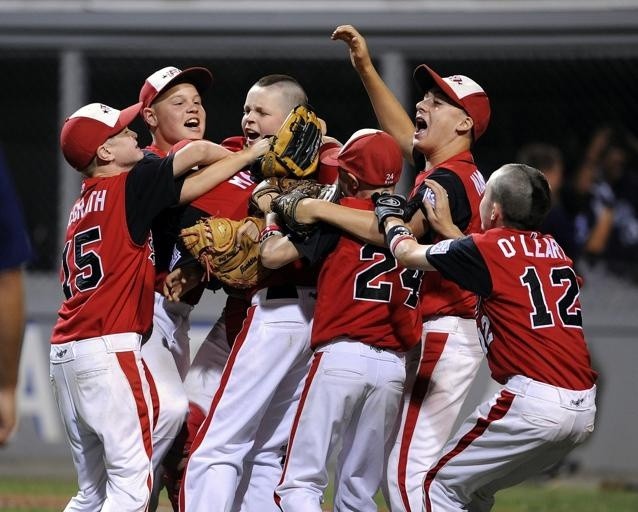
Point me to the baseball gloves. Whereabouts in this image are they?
[181,216,267,288]
[250,181,341,239]
[261,105,327,178]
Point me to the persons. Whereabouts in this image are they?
[517,123,638,274]
[48,24,601,511]
[0,151,35,446]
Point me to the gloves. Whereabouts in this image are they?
[270,189,321,238]
[372,190,416,226]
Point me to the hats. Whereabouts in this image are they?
[139,66,214,121]
[60,101,144,172]
[319,128,403,186]
[413,64,491,142]
